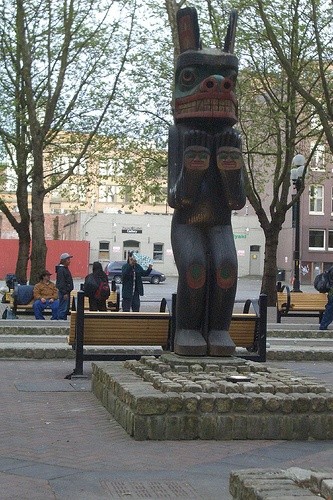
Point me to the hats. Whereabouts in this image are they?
[6,273,17,283]
[60,253,73,259]
[41,270,53,276]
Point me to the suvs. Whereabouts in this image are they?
[105,260,167,284]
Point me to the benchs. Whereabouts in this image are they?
[64,291,268,380]
[276,282,329,324]
[10,281,121,320]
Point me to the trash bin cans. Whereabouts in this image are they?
[277,270,285,281]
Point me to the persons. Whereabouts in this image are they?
[319,265,333,330]
[85,262,108,311]
[122,251,153,312]
[55,253,73,320]
[33,271,60,320]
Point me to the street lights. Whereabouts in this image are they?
[290,154,309,292]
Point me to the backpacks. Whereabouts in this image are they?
[92,272,111,301]
[314,266,333,293]
